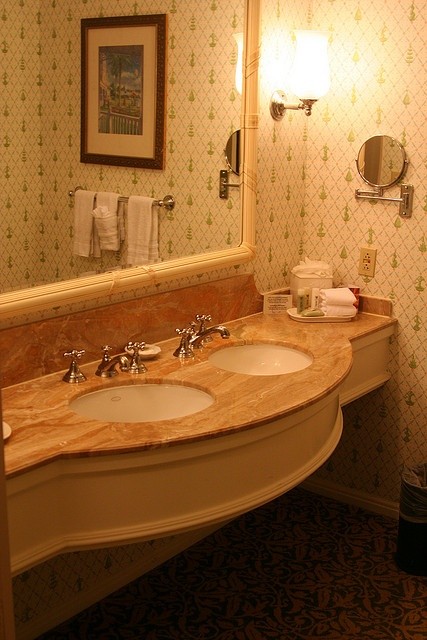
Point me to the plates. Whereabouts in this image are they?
[287,307,352,323]
[125,343,163,359]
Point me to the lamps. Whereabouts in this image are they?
[269,30,330,121]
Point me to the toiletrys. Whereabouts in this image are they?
[297,288,306,314]
[303,287,311,310]
[311,288,320,311]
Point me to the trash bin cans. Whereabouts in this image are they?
[393,464,427,576]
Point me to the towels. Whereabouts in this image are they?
[71,189,101,259]
[320,300,357,317]
[126,195,159,264]
[318,288,357,305]
[89,192,125,252]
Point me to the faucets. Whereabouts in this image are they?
[188,320,230,349]
[95,345,131,378]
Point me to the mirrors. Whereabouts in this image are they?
[217,128,241,199]
[0,0,260,317]
[354,134,410,190]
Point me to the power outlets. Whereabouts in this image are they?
[357,247,377,277]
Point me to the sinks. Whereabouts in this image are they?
[208,339,314,376]
[69,378,216,422]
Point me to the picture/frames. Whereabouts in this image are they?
[79,13,169,169]
